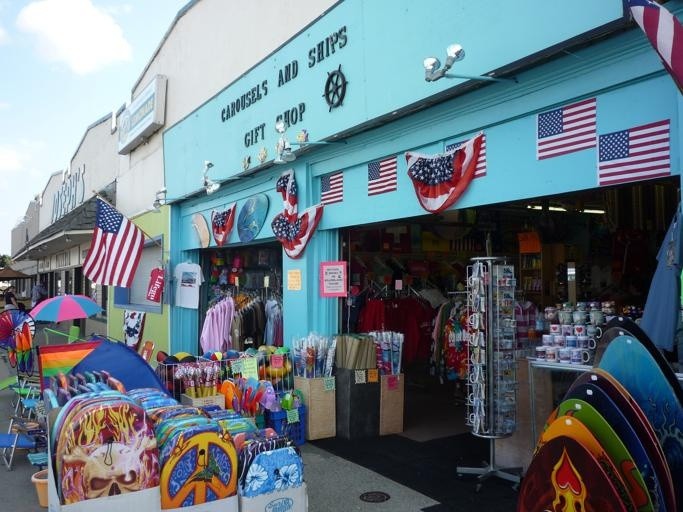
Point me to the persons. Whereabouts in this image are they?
[4,285,18,310]
[30,281,46,308]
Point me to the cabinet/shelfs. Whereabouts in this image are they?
[344,219,520,261]
[518,245,555,311]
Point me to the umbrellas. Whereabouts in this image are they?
[0,267,31,288]
[28,293,106,323]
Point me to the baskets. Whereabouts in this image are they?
[268,406,305,447]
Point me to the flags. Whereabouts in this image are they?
[595,118,671,187]
[318,172,342,205]
[81,191,145,289]
[535,98,598,161]
[624,0,683,93]
[366,157,397,198]
[445,134,486,179]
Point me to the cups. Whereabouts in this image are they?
[534,300,616,365]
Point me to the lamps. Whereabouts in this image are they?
[422,43,518,87]
[272,136,348,166]
[146,187,197,214]
[199,160,255,195]
